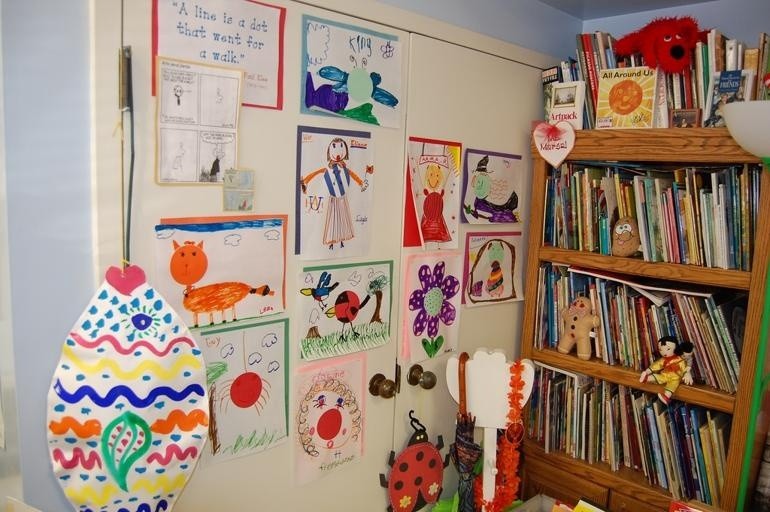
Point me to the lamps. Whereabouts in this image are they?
[718,100,770,511]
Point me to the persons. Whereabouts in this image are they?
[638,336,694,404]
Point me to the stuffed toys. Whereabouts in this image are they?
[613,16,711,73]
[379,410,450,511]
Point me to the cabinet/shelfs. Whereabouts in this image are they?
[514,120,769,512]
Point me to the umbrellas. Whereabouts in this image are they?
[448,352,482,511]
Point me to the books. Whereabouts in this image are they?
[534,262,747,395]
[542,161,763,271]
[526,361,731,508]
[541,28,769,129]
